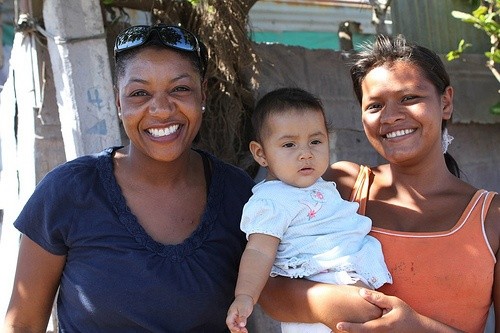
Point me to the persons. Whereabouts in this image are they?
[225,88,391,333]
[2,24,256,333]
[257,33,500,333]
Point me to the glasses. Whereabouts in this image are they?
[112,25,205,68]
